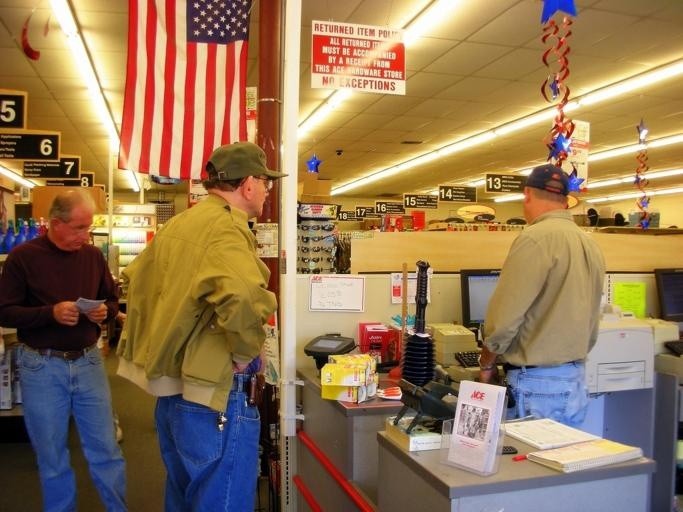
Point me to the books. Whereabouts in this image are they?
[502,414,600,451]
[526,440,643,473]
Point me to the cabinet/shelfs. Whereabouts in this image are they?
[94,213,156,303]
[0,254,23,416]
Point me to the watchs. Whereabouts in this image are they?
[478,355,494,372]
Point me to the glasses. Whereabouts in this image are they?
[54,215,95,234]
[252,177,273,191]
[297,223,336,274]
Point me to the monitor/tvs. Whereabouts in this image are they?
[460,268,501,327]
[654,268,683,321]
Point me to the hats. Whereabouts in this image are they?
[524,163,569,195]
[206,142,289,182]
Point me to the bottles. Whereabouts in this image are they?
[0,216,48,254]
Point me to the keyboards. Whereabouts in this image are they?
[454,350,503,366]
[665,340,683,356]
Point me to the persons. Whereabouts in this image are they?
[0,184,133,511]
[479,159,604,431]
[122,141,276,511]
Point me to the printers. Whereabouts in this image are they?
[582,319,655,395]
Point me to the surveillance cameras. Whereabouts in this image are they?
[336,150,343,157]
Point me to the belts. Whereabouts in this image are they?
[505,359,582,369]
[26,343,97,359]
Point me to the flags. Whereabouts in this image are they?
[117,2,254,179]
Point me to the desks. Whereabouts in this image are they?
[439,364,504,393]
